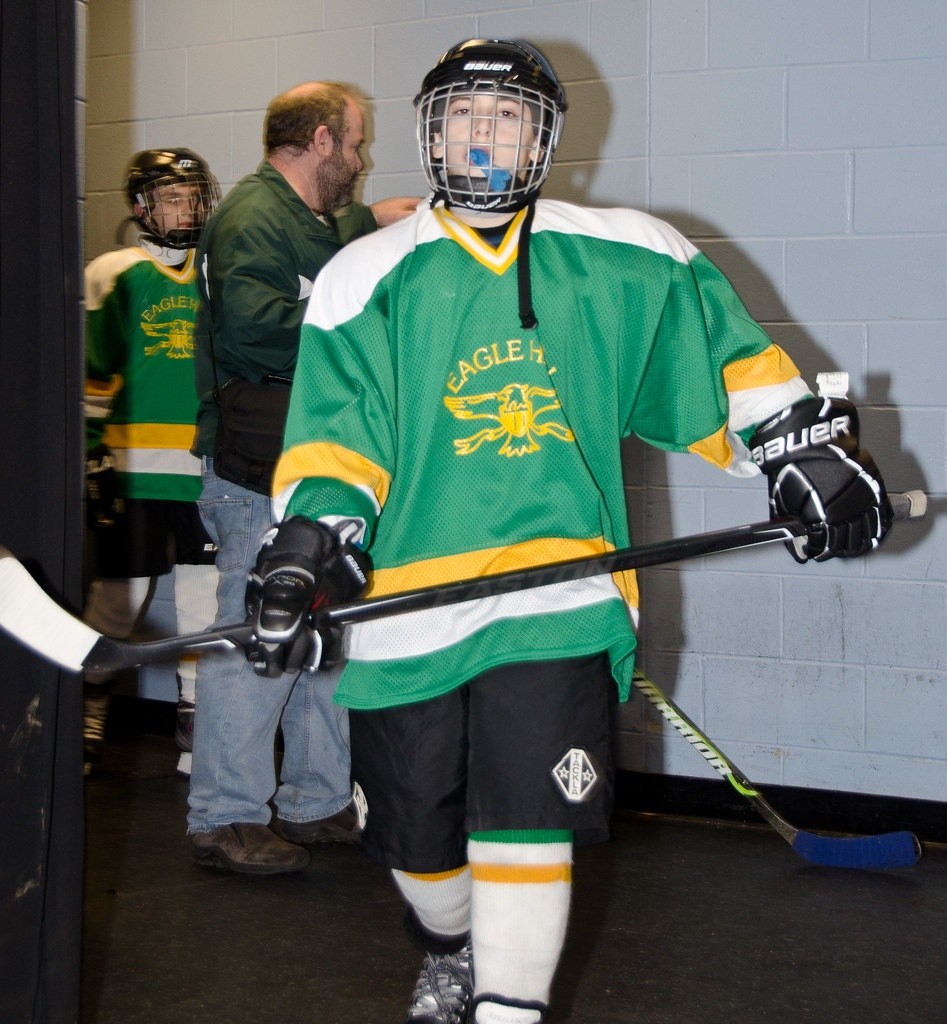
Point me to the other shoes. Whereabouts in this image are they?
[406,930,473,1024]
[278,811,367,853]
[173,700,196,775]
[83,691,110,778]
[472,993,548,1024]
[183,822,310,874]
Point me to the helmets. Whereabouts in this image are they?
[413,40,570,330]
[115,148,219,250]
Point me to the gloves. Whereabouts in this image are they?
[83,443,125,532]
[244,515,371,678]
[748,371,895,565]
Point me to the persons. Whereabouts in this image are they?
[246,39,892,1024]
[81,148,218,794]
[187,81,425,878]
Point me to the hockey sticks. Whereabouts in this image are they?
[0,488,929,688]
[632,663,925,873]
